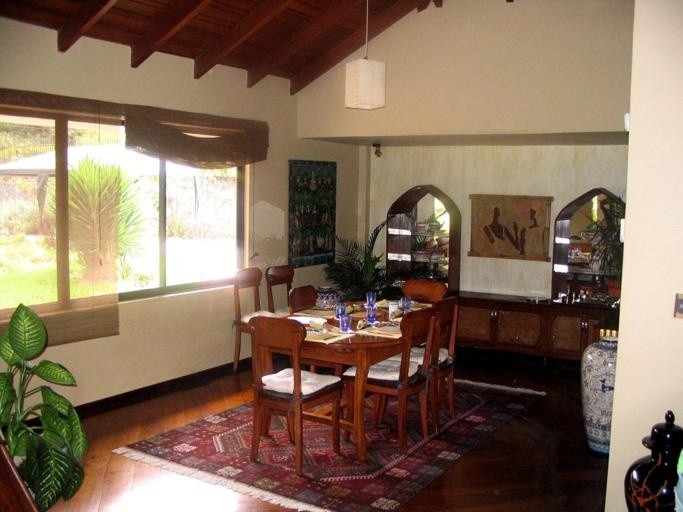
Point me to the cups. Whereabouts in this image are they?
[366,292,376,310]
[339,314,351,333]
[335,303,346,319]
[367,307,376,324]
[400,297,411,312]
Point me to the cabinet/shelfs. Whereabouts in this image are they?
[547,308,605,365]
[456,300,545,354]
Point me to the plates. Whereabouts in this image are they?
[287,316,328,325]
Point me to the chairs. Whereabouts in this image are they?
[338,305,436,453]
[399,278,449,305]
[387,295,461,443]
[265,264,295,313]
[250,316,343,474]
[288,284,342,377]
[233,268,277,373]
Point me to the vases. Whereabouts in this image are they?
[580,335,619,454]
[316,287,341,309]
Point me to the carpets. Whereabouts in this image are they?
[112,380,547,511]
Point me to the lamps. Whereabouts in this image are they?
[345,0,385,111]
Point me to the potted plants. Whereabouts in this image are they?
[411,236,433,259]
[44,151,149,284]
[570,191,625,299]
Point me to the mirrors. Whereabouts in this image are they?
[385,184,461,295]
[552,187,624,308]
